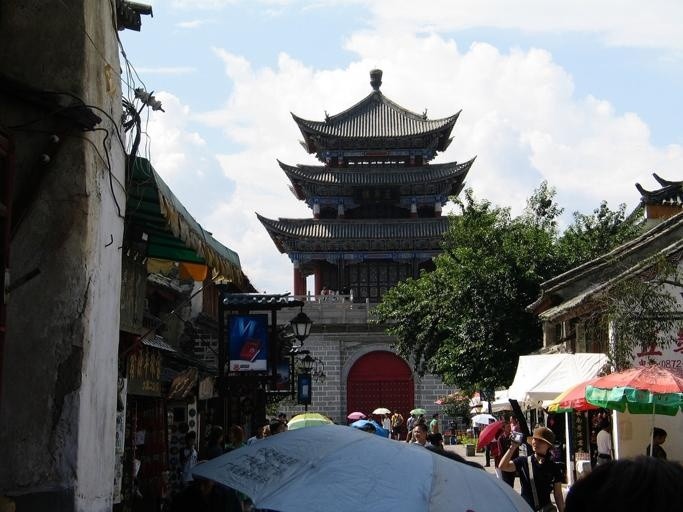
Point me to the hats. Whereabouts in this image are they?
[532,427,555,446]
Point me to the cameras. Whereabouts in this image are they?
[511,431,525,444]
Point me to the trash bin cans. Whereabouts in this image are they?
[467,445,475,456]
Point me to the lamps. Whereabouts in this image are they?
[301,354,314,372]
[289,306,312,346]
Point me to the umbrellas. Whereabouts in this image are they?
[409,408,426,415]
[372,407,390,419]
[351,418,387,437]
[286,413,335,431]
[189,423,537,512]
[347,411,365,420]
[547,364,683,458]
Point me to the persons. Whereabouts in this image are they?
[563,455,683,512]
[358,409,445,450]
[646,426,669,460]
[168,408,289,509]
[593,420,613,465]
[464,406,566,512]
